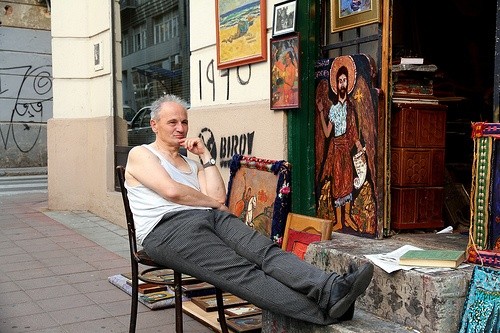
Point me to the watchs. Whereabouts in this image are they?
[203,157,215,168]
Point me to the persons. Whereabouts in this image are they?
[125,93,374,327]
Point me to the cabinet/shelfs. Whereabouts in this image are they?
[390,102,449,230]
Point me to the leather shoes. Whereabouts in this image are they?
[327,261,374,319]
[337,260,358,321]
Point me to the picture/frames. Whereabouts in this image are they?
[272,0,298,39]
[270,32,302,110]
[225,153,292,249]
[215,0,267,70]
[182,282,262,333]
[330,0,381,33]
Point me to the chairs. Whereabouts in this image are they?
[115,165,229,333]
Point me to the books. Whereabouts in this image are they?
[399,249,466,268]
